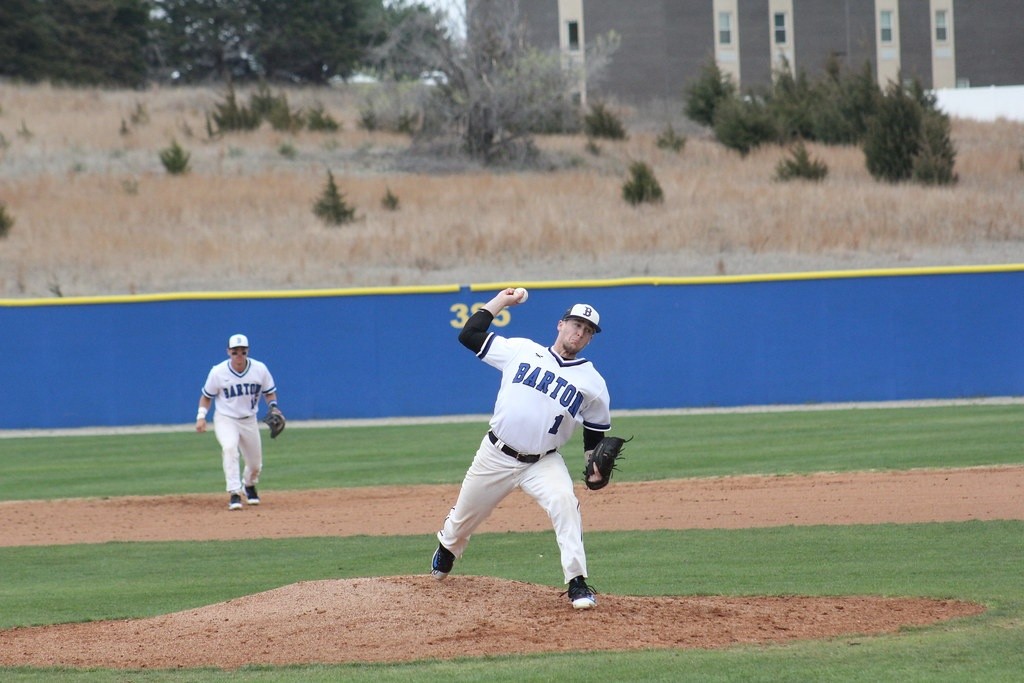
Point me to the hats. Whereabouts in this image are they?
[563,303,602,333]
[228,333,249,349]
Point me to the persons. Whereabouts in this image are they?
[195,333,281,511]
[431,289,612,609]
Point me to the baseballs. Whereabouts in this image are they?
[514,288,528,304]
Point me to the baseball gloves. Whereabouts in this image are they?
[263,408,286,438]
[583,437,624,490]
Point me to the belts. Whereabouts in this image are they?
[489,431,557,463]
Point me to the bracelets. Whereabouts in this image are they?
[196,414,206,419]
[198,407,208,415]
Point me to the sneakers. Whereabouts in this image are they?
[242,485,261,504]
[430,542,456,580]
[559,575,597,610]
[228,493,243,511]
